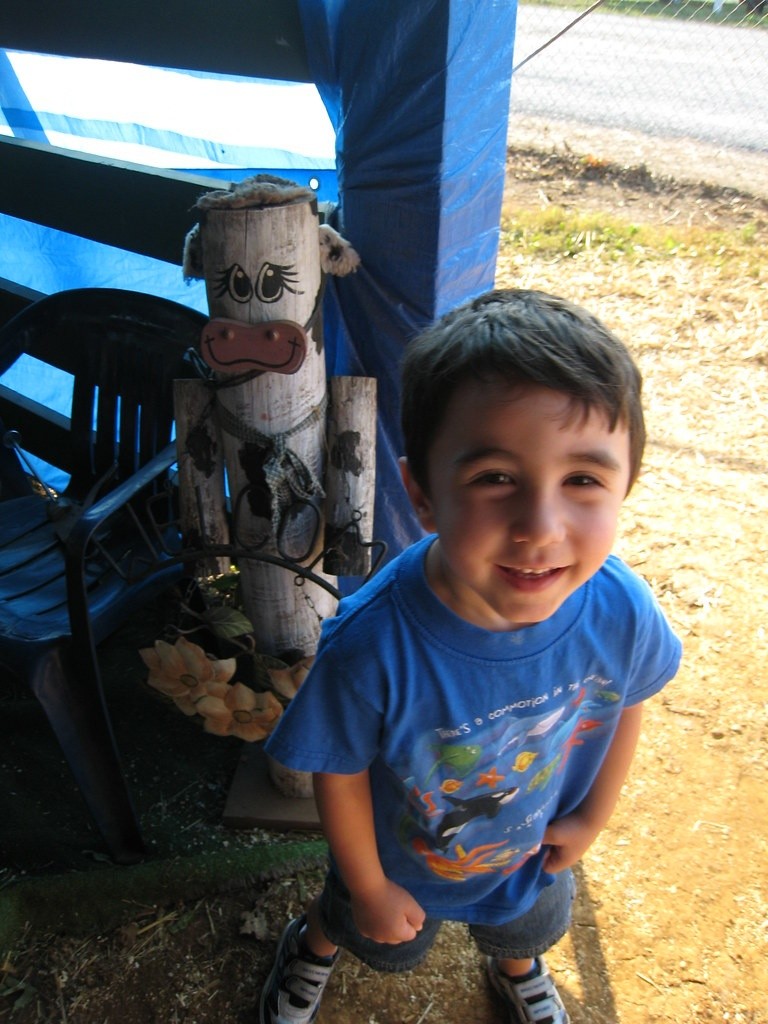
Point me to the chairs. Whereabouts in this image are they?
[0,288,262,861]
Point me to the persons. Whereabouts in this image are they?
[254,290,679,1024]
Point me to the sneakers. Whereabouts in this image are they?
[259,913,342,1024]
[487,956,570,1024]
